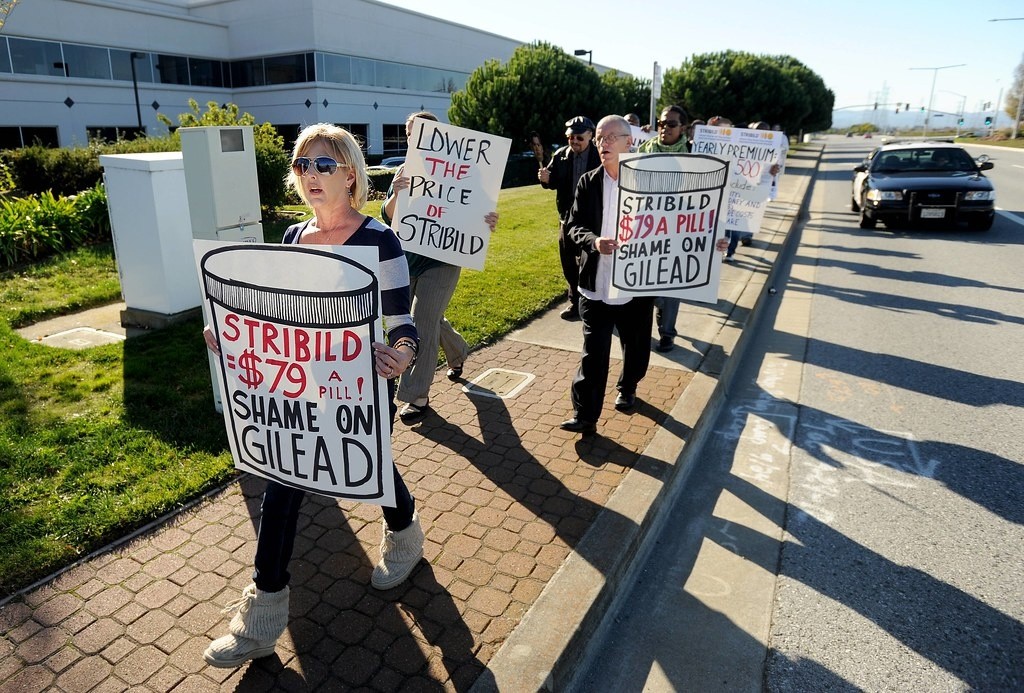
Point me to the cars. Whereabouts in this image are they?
[849,138,996,235]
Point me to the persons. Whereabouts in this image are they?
[202,124,424,671]
[540,104,789,430]
[381,112,499,428]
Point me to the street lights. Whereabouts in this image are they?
[574,49,593,64]
[909,63,965,139]
[130,51,146,139]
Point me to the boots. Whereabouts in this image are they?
[202,582,289,669]
[371,509,425,590]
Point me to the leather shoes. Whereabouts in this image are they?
[614,391,636,410]
[560,415,597,434]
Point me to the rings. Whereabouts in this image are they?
[385,369,394,378]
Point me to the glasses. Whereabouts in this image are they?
[592,134,629,146]
[657,120,682,128]
[569,134,588,141]
[292,157,349,177]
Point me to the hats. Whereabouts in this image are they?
[688,120,705,129]
[564,116,595,135]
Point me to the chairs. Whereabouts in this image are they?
[881,150,959,170]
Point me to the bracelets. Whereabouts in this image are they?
[394,339,417,369]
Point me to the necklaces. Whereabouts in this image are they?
[312,208,352,244]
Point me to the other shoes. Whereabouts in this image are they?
[399,396,429,426]
[724,253,735,262]
[742,239,753,247]
[725,230,731,238]
[656,337,674,353]
[560,304,583,321]
[447,361,464,380]
